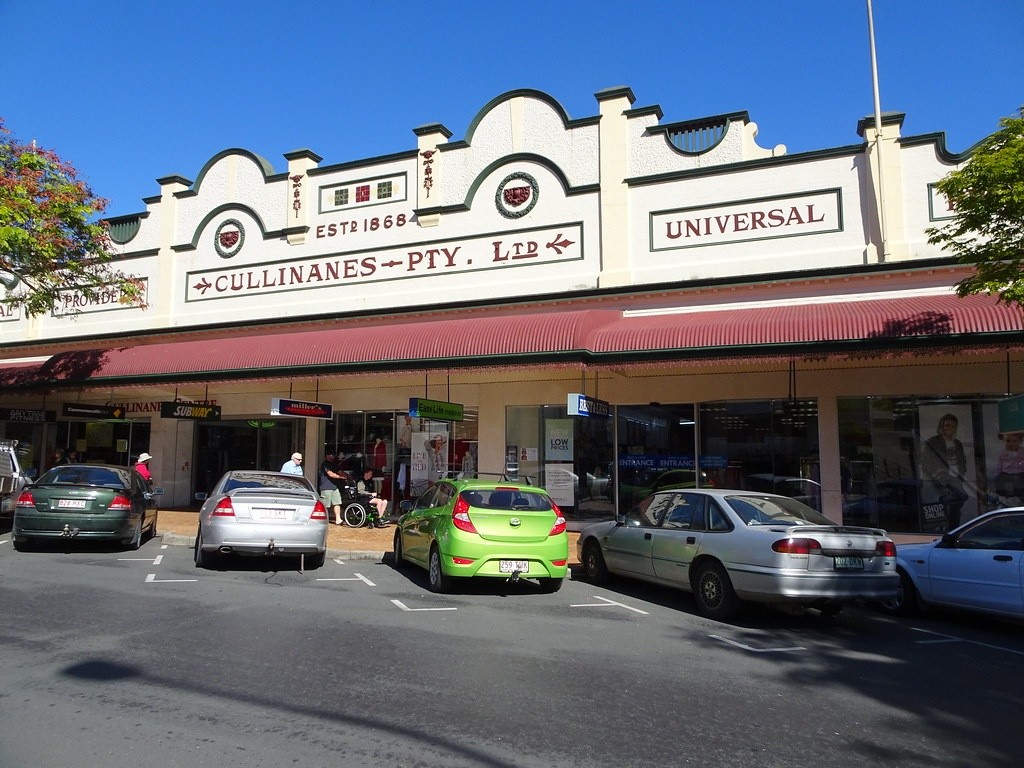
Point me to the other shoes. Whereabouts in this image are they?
[372,518,390,527]
[335,520,347,525]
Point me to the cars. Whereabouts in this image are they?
[740,473,821,509]
[519,465,610,500]
[11,462,166,552]
[194,469,329,571]
[877,506,1024,618]
[576,488,902,621]
[393,469,569,594]
[632,468,719,507]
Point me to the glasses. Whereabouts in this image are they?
[296,458,302,461]
[73,454,77,456]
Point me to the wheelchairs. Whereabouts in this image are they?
[344,477,391,528]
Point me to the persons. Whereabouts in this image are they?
[320,449,347,524]
[136,453,153,479]
[65,448,78,464]
[994,433,1024,496]
[357,467,388,519]
[462,451,475,478]
[52,449,64,466]
[923,414,966,532]
[424,434,444,489]
[400,416,414,449]
[281,453,303,476]
[373,438,386,496]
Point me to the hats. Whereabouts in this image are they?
[137,453,152,464]
[327,449,337,455]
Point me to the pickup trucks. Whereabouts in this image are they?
[0,440,33,516]
